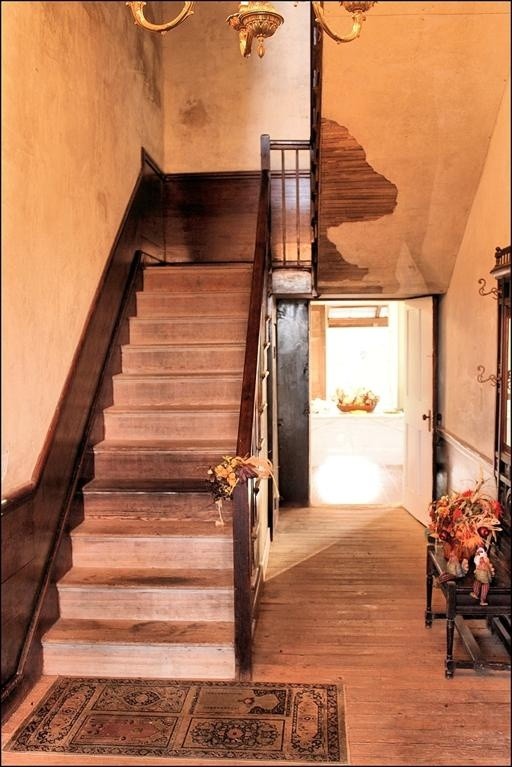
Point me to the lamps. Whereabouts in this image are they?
[118,1,383,59]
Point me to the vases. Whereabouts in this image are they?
[443,540,471,560]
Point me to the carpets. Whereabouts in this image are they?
[0,672,355,765]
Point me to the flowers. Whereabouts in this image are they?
[207,451,284,530]
[426,462,504,561]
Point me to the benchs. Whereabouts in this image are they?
[425,542,512,680]
